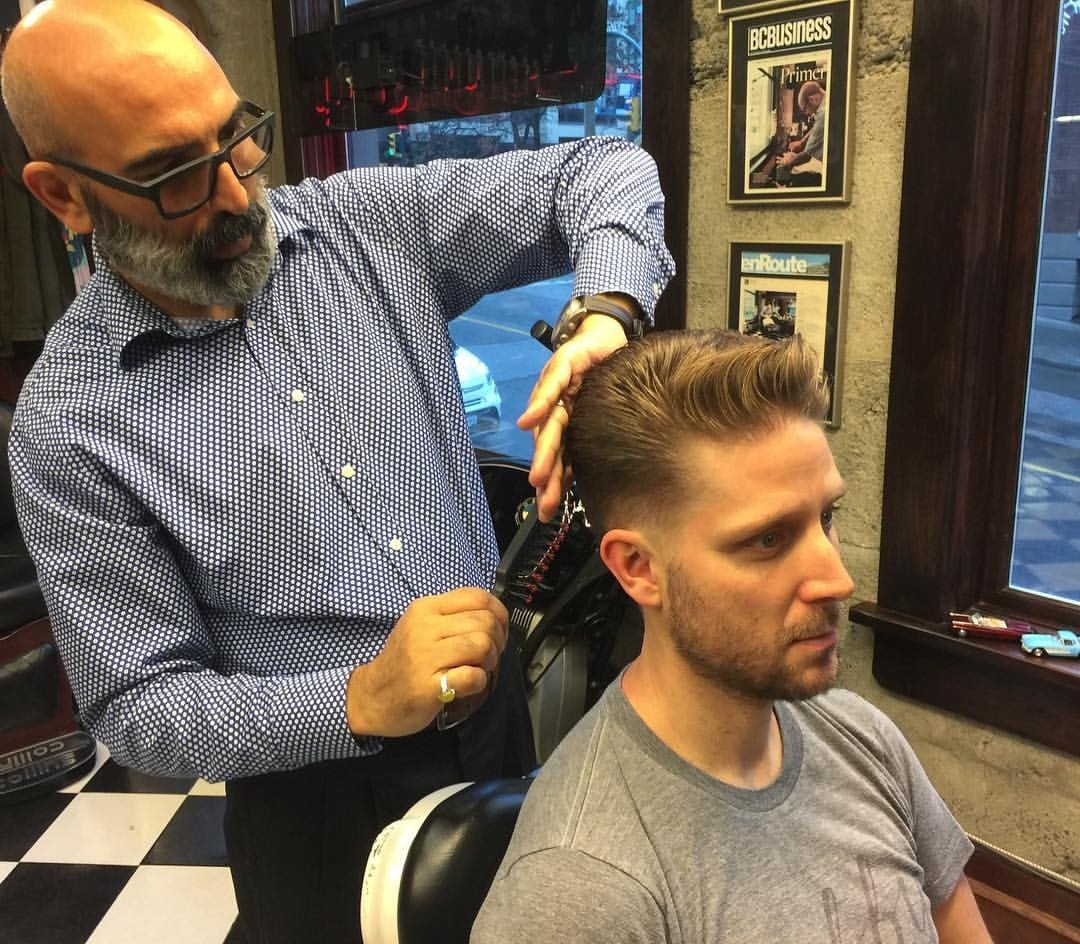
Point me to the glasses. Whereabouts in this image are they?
[47,98,277,222]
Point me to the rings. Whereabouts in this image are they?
[439,671,454,704]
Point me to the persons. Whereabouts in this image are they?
[469,327,1000,944]
[0,1,675,941]
[776,80,826,168]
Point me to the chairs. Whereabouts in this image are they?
[360,769,538,942]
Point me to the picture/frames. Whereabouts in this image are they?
[725,239,855,433]
[727,1,857,211]
[715,1,800,15]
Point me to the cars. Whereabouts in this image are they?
[449,340,506,449]
